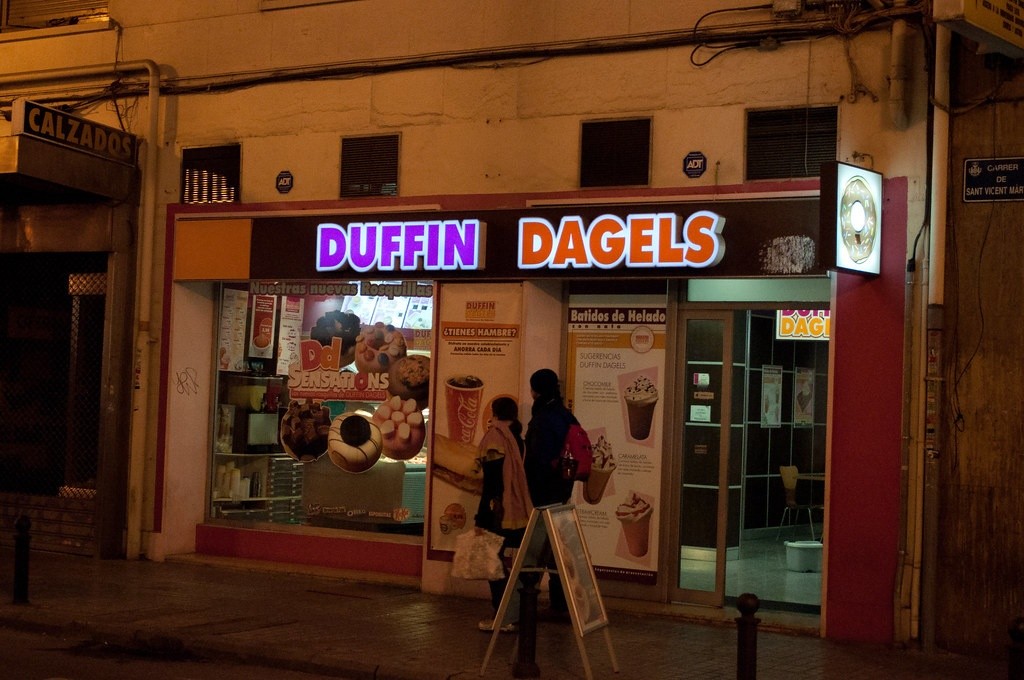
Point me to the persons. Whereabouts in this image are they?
[524,369,571,628]
[475,397,525,631]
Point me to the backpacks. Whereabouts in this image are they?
[551,420,592,482]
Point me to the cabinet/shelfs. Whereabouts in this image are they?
[211,446,305,527]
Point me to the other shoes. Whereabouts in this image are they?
[477,618,515,633]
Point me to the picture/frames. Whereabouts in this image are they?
[546,503,610,638]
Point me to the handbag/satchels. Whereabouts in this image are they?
[451,528,507,581]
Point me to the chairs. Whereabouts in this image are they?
[775,465,826,545]
